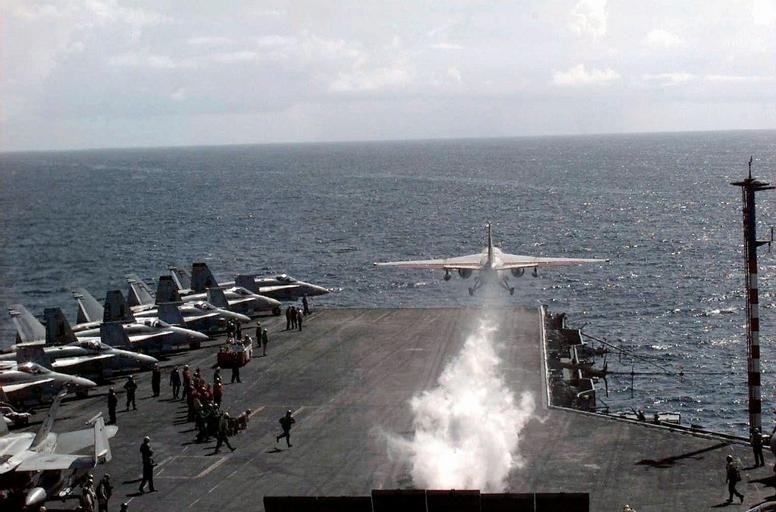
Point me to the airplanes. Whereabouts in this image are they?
[215,272,330,308]
[54,286,211,357]
[114,271,253,339]
[1,379,119,506]
[1,306,160,377]
[179,263,284,318]
[373,222,610,295]
[1,361,98,407]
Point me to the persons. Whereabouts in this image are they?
[262,328,269,355]
[96,473,114,512]
[724,454,745,503]
[750,427,765,467]
[286,306,303,331]
[82,474,96,512]
[277,410,295,447]
[139,436,158,493]
[152,363,161,397]
[170,366,181,400]
[124,375,138,411]
[182,365,237,453]
[226,316,242,339]
[108,387,118,424]
[238,409,252,427]
[302,293,310,315]
[219,333,252,352]
[256,321,262,347]
